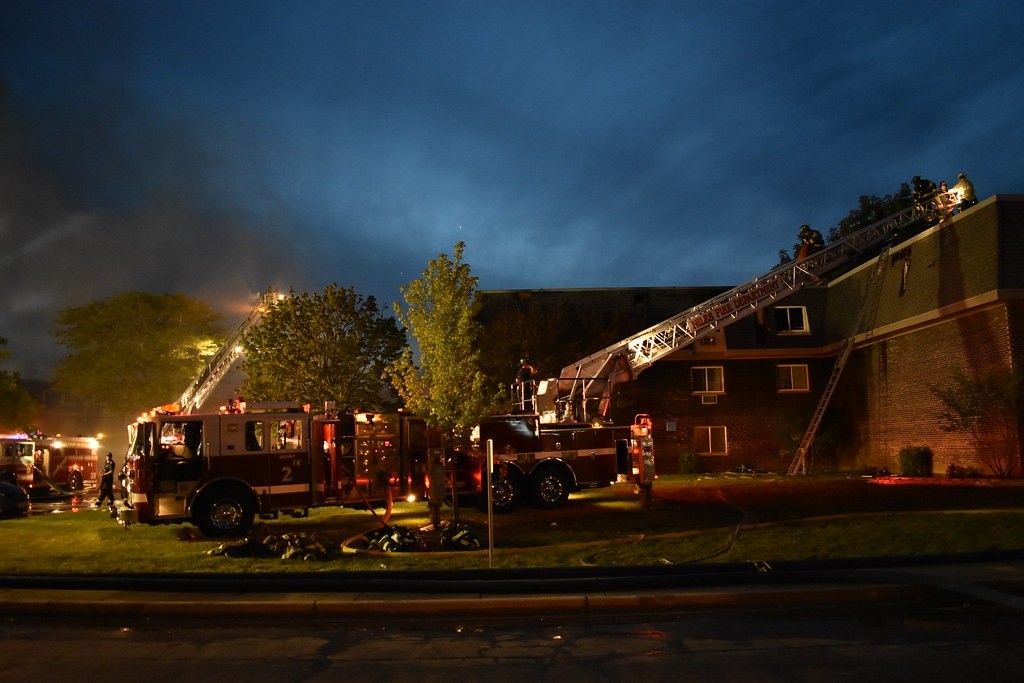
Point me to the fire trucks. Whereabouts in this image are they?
[128,286,286,447]
[0,432,100,492]
[111,173,976,539]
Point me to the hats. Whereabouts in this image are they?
[107,452,112,456]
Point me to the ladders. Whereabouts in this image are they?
[785,242,893,477]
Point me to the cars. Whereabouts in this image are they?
[0,480,31,514]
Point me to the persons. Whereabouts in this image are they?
[800,224,830,273]
[94,452,116,506]
[34,458,44,488]
[119,460,130,499]
[931,173,977,223]
[516,356,537,412]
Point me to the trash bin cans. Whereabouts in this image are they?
[899,446,934,477]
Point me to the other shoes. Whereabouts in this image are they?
[107,501,113,504]
[95,502,101,506]
